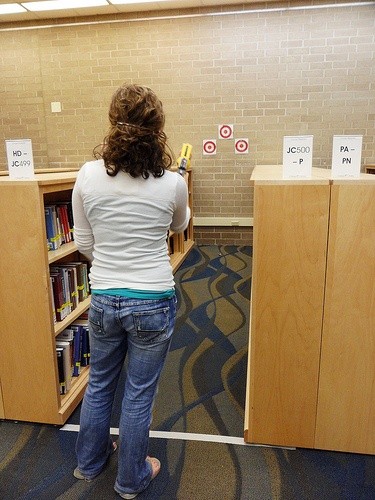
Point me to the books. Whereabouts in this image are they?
[44,199,77,251]
[48,259,91,325]
[53,309,92,395]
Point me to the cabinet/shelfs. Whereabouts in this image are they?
[0,168,198,424]
[244,165,375,455]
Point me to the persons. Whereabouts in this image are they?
[69,82,193,499]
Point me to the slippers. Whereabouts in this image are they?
[73,433,118,480]
[113,455,161,499]
